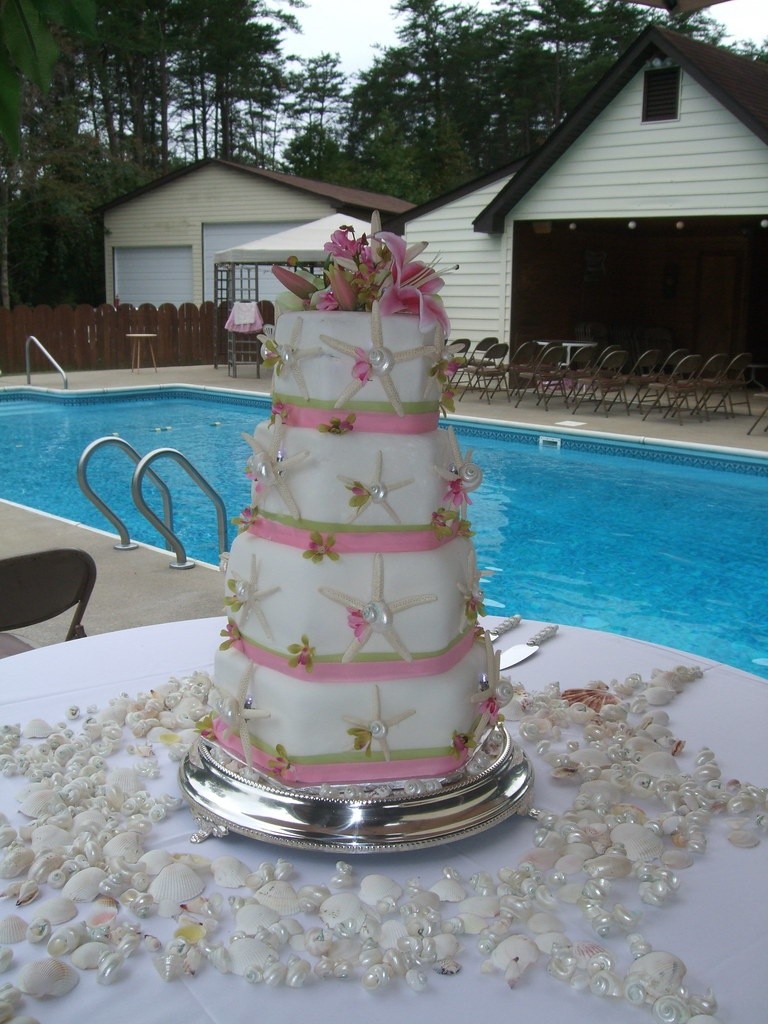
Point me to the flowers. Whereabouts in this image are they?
[272,211,460,335]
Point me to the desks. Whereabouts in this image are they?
[126,334,158,374]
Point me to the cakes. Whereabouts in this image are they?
[208,309,507,793]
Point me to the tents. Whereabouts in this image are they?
[213,213,386,370]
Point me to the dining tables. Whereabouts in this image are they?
[0,611,768,1024]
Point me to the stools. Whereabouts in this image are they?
[227,330,261,380]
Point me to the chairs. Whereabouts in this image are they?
[443,337,768,435]
[0,547,98,641]
